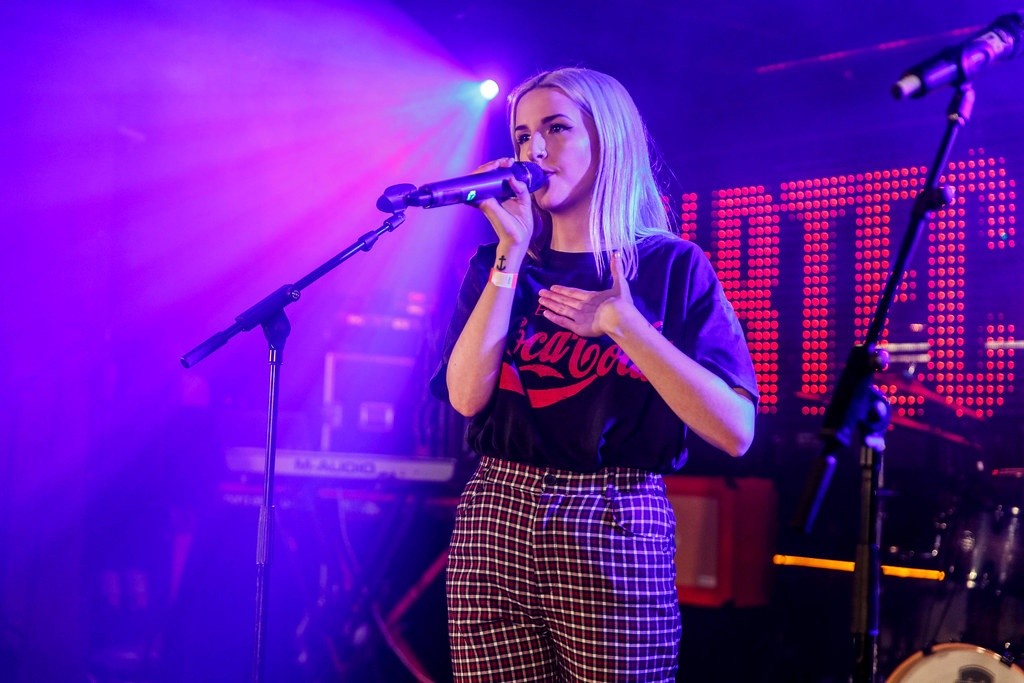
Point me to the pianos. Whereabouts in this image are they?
[215,443,461,501]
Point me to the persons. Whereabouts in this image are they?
[429,67,758,683]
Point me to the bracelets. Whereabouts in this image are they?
[489,268,518,288]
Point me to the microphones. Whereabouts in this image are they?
[889,12,1024,102]
[406,161,545,209]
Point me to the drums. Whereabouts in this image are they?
[888,464,1024,683]
[660,473,778,608]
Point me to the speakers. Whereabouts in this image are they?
[662,474,775,612]
